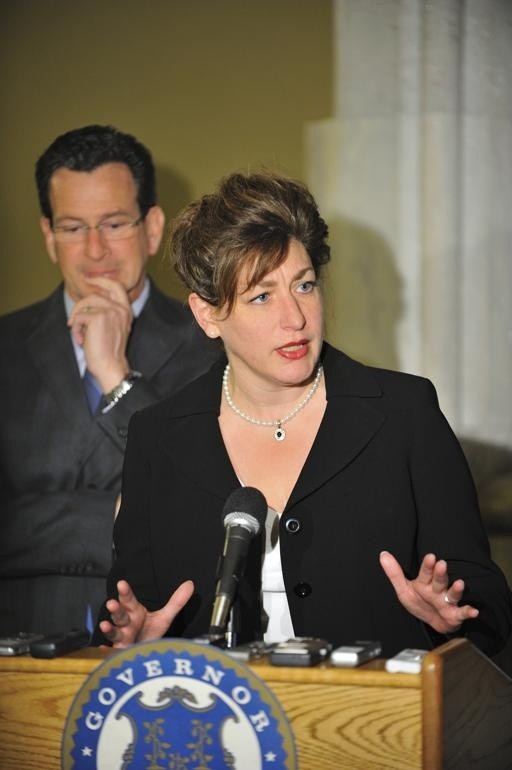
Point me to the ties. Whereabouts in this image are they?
[81,367,105,417]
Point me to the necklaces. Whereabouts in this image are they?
[222,360,323,441]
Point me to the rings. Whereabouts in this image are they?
[444,592,458,606]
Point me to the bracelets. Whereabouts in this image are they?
[99,371,141,411]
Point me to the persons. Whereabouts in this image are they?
[89,167,511,681]
[0,122,227,649]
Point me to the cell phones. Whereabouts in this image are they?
[29,629,90,657]
[330,638,383,669]
[0,632,47,656]
[385,648,429,674]
[269,637,332,667]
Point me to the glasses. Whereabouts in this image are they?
[48,212,149,246]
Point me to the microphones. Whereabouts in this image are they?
[206,486,268,645]
